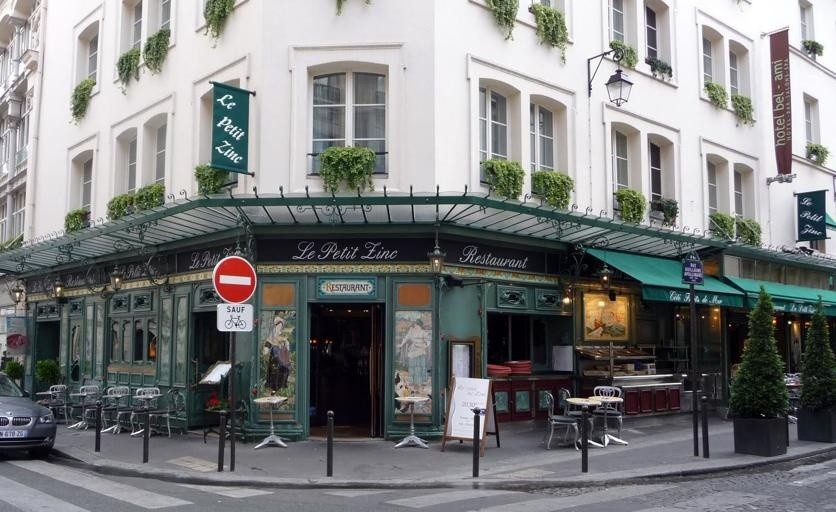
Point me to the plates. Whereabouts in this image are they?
[487,360,534,377]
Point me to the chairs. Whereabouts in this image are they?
[34,385,186,441]
[545,386,628,450]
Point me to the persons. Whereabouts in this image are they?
[396,316,431,388]
[260,315,293,397]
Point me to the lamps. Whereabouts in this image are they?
[598,247,614,289]
[12,260,123,305]
[425,222,446,274]
[587,48,633,108]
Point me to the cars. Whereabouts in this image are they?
[1,370,57,458]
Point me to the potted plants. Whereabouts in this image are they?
[727,287,835,458]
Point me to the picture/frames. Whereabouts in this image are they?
[581,292,631,343]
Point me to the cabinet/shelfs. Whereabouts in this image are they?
[536,381,574,421]
[624,390,653,415]
[493,382,534,422]
[655,389,680,412]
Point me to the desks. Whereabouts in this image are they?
[200,399,241,444]
[253,396,290,450]
[395,397,430,449]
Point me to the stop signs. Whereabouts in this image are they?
[211,256,257,302]
